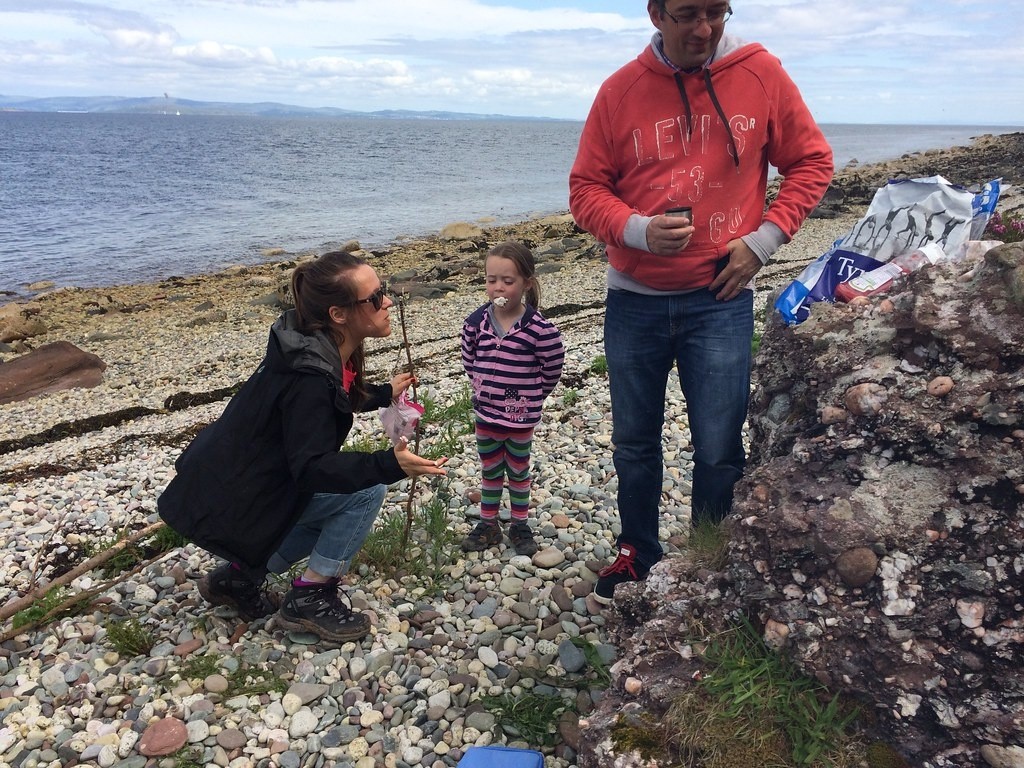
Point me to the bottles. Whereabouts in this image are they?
[836,242,946,304]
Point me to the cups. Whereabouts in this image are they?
[665,207,691,229]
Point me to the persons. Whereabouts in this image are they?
[462,242,564,555]
[844,202,965,251]
[569,1,833,605]
[157,252,449,641]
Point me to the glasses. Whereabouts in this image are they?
[338,280,386,310]
[656,1,734,29]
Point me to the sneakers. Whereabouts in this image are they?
[277,575,372,643]
[594,541,651,605]
[508,524,537,556]
[197,560,275,623]
[463,521,503,552]
